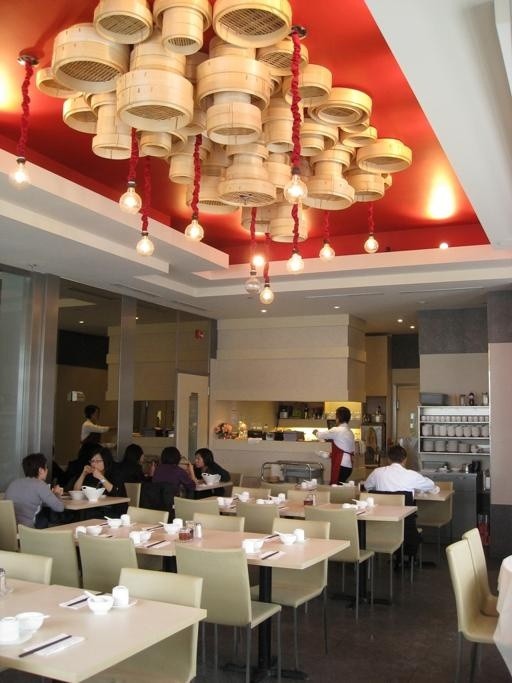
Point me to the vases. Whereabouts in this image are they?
[218,433,227,439]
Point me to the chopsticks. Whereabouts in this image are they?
[261,550,280,561]
[147,525,164,530]
[19,635,73,659]
[146,539,168,549]
[266,533,278,540]
[356,510,366,516]
[67,590,106,607]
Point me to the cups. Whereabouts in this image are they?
[266,432,274,439]
[75,526,87,538]
[343,479,365,491]
[295,529,305,542]
[420,414,488,421]
[342,497,375,509]
[129,531,141,545]
[0,616,18,639]
[111,585,129,607]
[173,518,203,542]
[299,478,318,487]
[121,514,130,525]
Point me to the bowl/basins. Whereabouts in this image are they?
[87,526,103,536]
[86,596,113,613]
[164,524,176,535]
[141,531,152,541]
[202,472,222,487]
[215,491,286,510]
[107,519,122,528]
[69,486,105,502]
[243,538,264,551]
[470,449,480,453]
[280,534,297,546]
[15,612,44,630]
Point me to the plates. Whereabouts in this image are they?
[129,597,138,607]
[1,636,33,647]
[421,438,470,452]
[451,468,462,472]
[421,424,490,438]
[476,445,490,448]
[370,414,385,423]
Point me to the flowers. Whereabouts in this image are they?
[214,423,232,435]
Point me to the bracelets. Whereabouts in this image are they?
[100,478,106,483]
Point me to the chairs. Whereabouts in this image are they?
[0,551,54,585]
[123,482,141,508]
[446,540,499,683]
[17,522,81,588]
[249,517,331,670]
[117,566,204,610]
[76,531,138,593]
[1,500,20,553]
[460,527,502,619]
[192,512,246,533]
[173,480,454,624]
[126,506,170,525]
[174,543,283,682]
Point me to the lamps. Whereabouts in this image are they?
[318,208,336,264]
[282,29,310,205]
[363,200,379,254]
[246,207,263,296]
[135,163,155,259]
[259,233,276,305]
[12,62,35,187]
[185,134,206,243]
[119,127,143,216]
[288,203,306,275]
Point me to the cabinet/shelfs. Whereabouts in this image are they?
[417,406,490,455]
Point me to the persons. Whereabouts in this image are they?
[151,447,197,517]
[78,404,117,464]
[184,448,230,499]
[72,447,128,519]
[313,406,355,485]
[5,453,65,548]
[364,445,435,569]
[118,443,155,508]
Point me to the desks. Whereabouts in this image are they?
[494,555,512,677]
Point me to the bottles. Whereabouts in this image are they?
[459,394,466,406]
[1,568,7,593]
[303,404,322,418]
[482,392,488,406]
[363,413,369,424]
[467,393,475,406]
[376,406,382,415]
[304,491,318,507]
